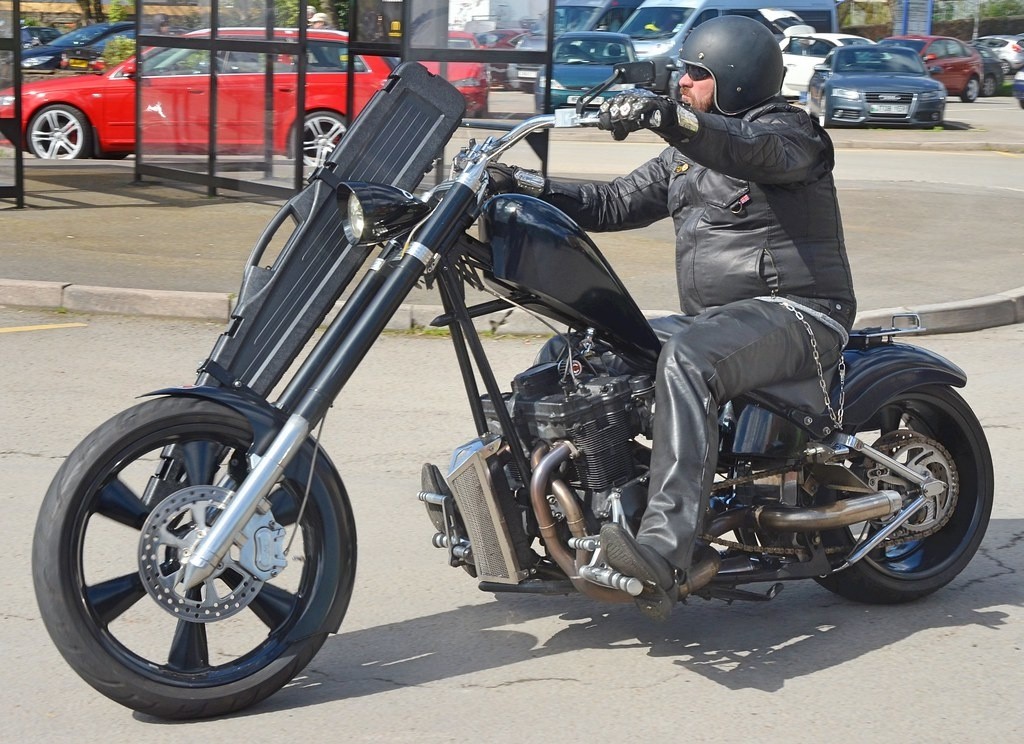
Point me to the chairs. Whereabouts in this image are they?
[604,43,628,63]
[560,45,585,60]
[840,51,866,70]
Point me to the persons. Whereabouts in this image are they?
[298,5,338,68]
[423,15,856,614]
[154,12,168,36]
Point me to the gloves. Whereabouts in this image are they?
[599,88,677,141]
[484,162,516,197]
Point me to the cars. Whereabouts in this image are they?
[9,17,200,90]
[0,12,404,161]
[413,0,1023,134]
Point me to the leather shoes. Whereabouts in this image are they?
[420,463,476,578]
[601,522,686,624]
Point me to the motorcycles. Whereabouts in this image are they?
[31,42,993,720]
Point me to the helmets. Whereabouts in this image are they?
[679,15,786,117]
